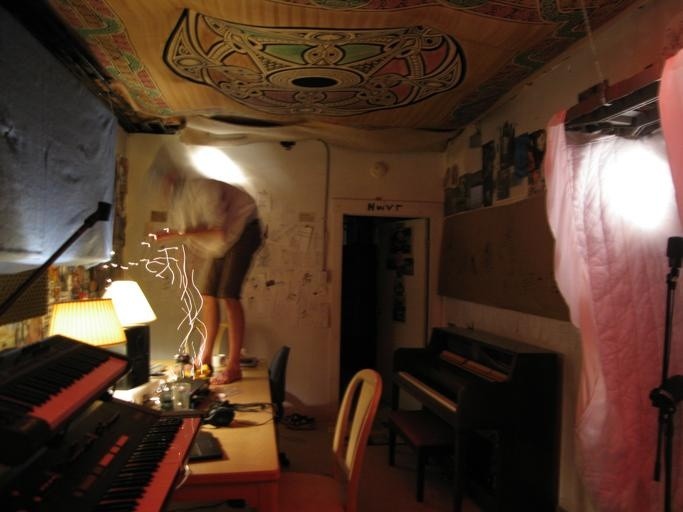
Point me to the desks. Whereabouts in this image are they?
[112,349,282,511]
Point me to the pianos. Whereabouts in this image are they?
[0,335,201,512]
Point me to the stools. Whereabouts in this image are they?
[387,406,466,511]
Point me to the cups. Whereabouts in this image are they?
[173,382,192,410]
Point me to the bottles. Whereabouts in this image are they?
[64,264,98,300]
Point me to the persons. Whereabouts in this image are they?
[145,149,262,387]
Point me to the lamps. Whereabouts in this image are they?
[45,298,128,351]
[101,281,156,391]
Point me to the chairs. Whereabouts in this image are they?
[269,347,290,419]
[271,369,382,512]
